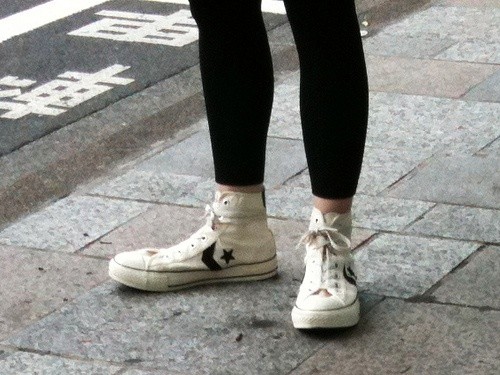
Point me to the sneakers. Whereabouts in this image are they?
[291,205,362,330]
[108,186,278,292]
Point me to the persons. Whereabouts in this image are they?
[106,0,368,331]
